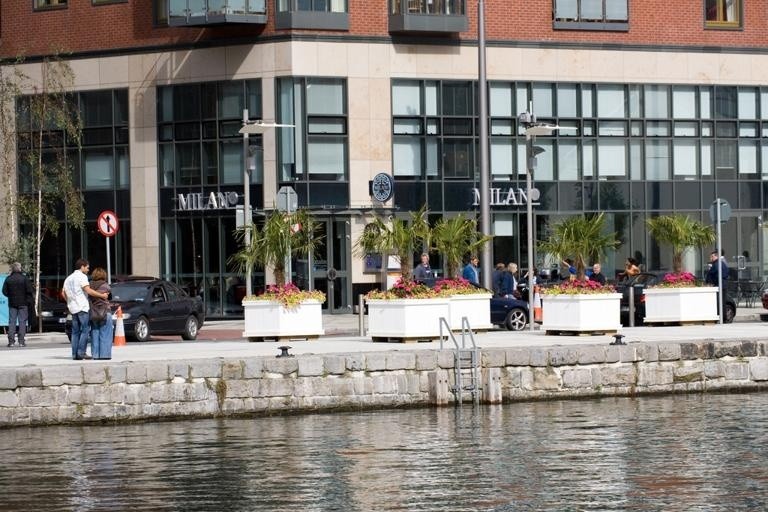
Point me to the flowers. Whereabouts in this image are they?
[363,275,450,300]
[432,274,494,296]
[242,280,327,314]
[543,277,618,298]
[647,272,704,288]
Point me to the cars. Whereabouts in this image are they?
[414,277,529,332]
[761,289,767,310]
[0,286,68,334]
[607,270,737,327]
[61,276,207,343]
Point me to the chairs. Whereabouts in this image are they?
[735,279,755,309]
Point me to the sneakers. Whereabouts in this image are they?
[8,343,27,347]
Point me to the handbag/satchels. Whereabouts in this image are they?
[89,300,107,321]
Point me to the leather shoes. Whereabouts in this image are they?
[77,354,92,360]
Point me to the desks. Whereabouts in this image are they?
[728,281,767,307]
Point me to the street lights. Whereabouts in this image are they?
[517,111,577,334]
[237,106,297,299]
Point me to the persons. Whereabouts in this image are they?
[499,262,519,300]
[413,251,434,287]
[737,251,753,298]
[715,248,727,265]
[490,263,505,296]
[587,262,606,288]
[523,265,539,288]
[2,260,34,347]
[85,267,114,360]
[59,258,110,362]
[462,256,481,289]
[617,258,640,279]
[707,252,728,325]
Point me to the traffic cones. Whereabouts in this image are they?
[533,283,541,320]
[111,305,128,346]
